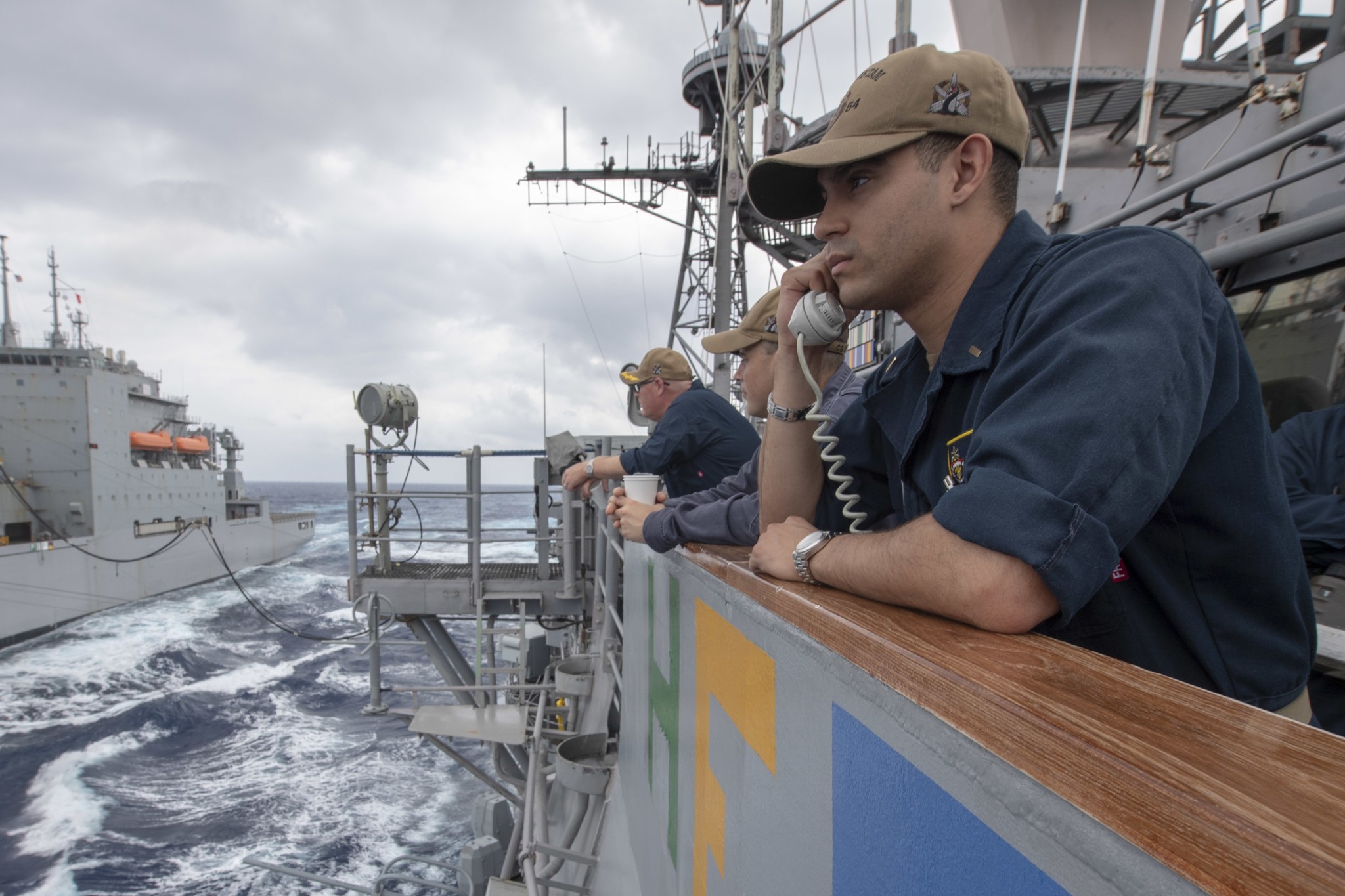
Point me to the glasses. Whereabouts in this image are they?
[634,379,670,392]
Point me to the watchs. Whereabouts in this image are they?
[586,459,595,478]
[793,531,843,584]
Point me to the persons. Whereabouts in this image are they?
[561,347,762,502]
[606,286,865,556]
[1270,403,1345,579]
[746,44,1321,725]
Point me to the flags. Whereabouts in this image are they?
[76,294,82,303]
[15,275,22,281]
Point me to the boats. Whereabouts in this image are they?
[345,0,1345,896]
[0,234,315,640]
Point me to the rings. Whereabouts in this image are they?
[563,483,568,487]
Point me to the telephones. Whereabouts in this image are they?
[789,287,846,354]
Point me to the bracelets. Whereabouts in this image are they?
[767,393,816,422]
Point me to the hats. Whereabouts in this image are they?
[701,284,849,355]
[619,347,696,384]
[747,39,1030,223]
